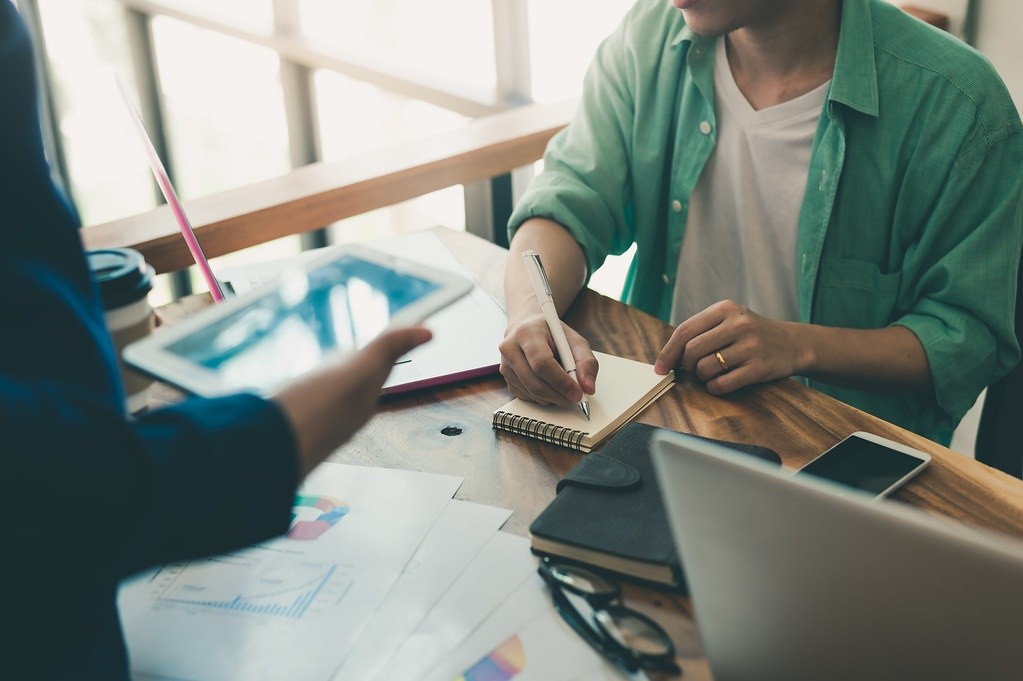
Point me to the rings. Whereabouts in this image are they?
[714,351,729,371]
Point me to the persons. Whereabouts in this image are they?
[498,0,1023,406]
[0,0,435,681]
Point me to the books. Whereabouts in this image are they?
[530,421,782,597]
[493,350,676,454]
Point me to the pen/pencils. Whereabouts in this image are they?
[521,248,591,420]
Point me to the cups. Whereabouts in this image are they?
[86,248,159,416]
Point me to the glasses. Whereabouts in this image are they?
[537,561,682,674]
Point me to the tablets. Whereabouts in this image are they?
[121,244,472,401]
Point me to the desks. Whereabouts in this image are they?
[120,224,1023,681]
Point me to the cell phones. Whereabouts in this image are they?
[791,430,933,498]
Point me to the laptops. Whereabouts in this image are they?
[653,430,1023,681]
